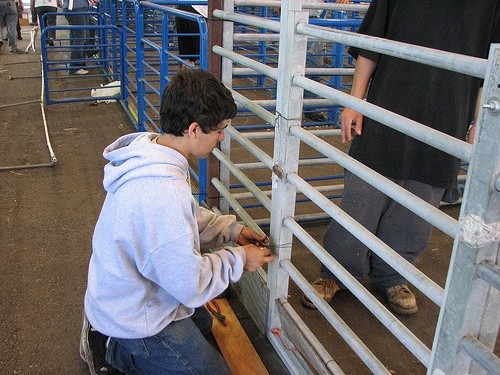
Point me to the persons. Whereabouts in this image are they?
[79,70,273,375]
[63,0,98,74]
[30,0,62,46]
[0,0,23,52]
[274,0,349,122]
[301,0,500,314]
[176,0,209,67]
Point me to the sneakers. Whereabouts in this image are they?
[384,282,418,314]
[303,276,340,309]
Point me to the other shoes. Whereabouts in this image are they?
[304,111,327,122]
[10,50,23,54]
[18,36,22,40]
[73,69,89,75]
[50,41,54,46]
[80,310,116,375]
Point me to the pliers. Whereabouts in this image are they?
[206,300,228,328]
